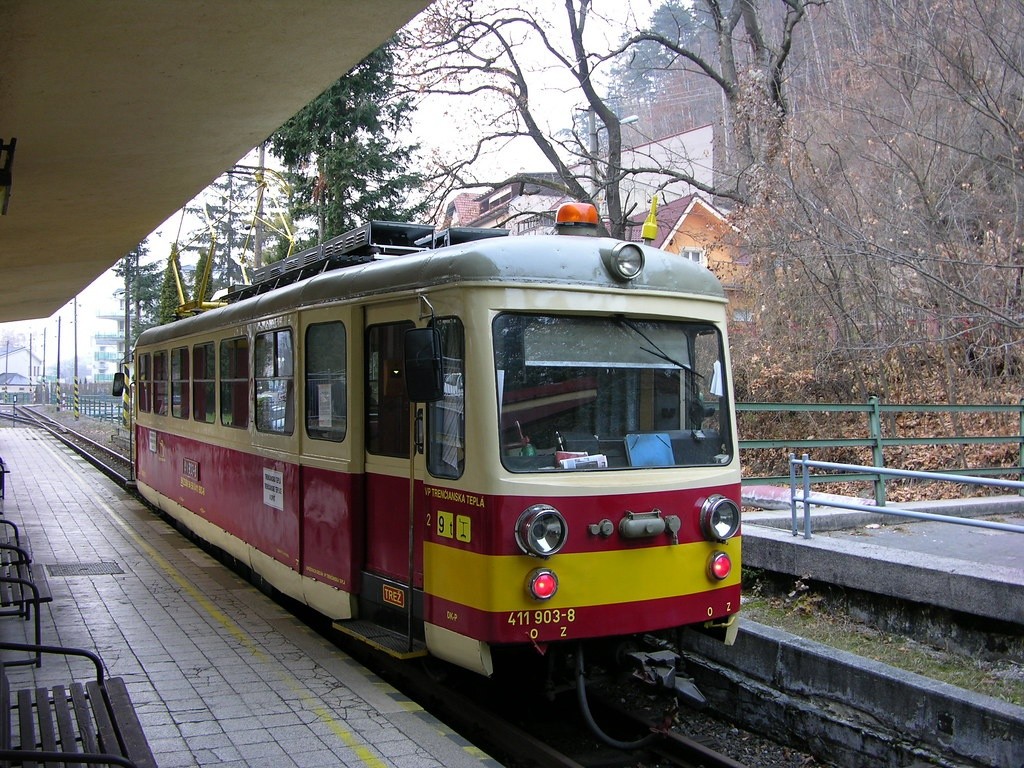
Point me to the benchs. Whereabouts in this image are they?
[0,457,10,500]
[0,640,160,768]
[0,518,54,644]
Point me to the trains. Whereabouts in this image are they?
[112,168,742,747]
